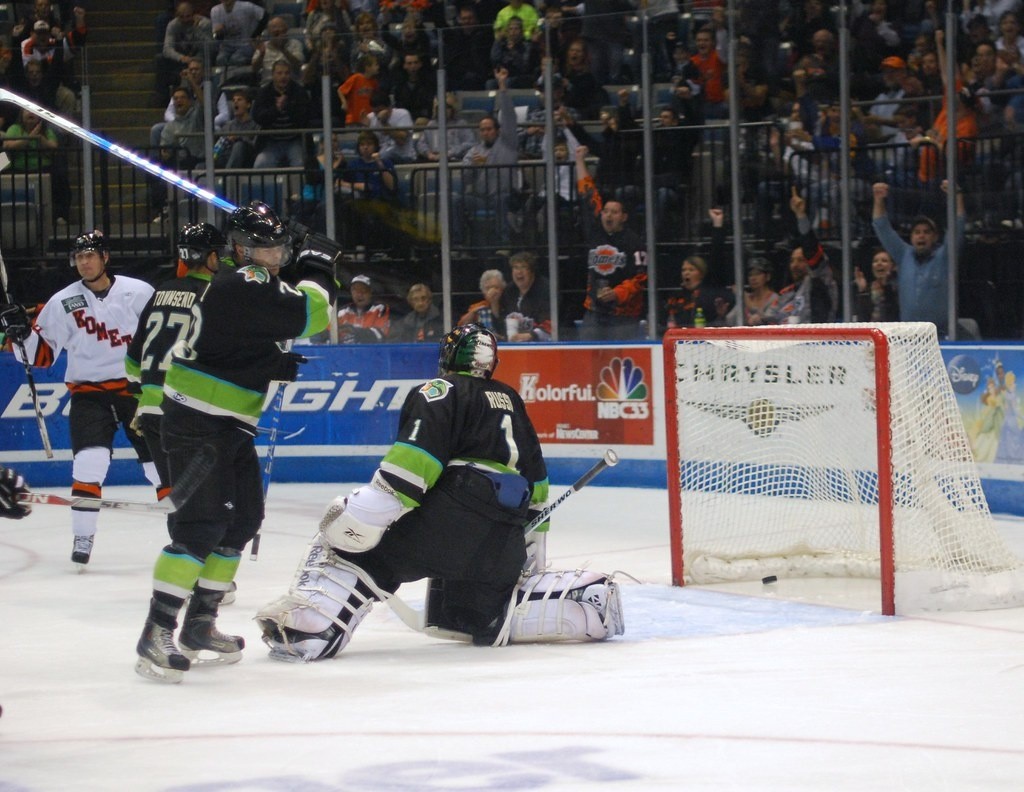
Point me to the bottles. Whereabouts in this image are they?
[694,307,706,328]
[213,136,230,160]
[666,310,676,330]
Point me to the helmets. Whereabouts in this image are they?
[225,200,293,269]
[175,222,228,271]
[69,229,110,261]
[438,322,499,380]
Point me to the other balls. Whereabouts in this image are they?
[761,576,777,584]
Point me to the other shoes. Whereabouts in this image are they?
[153,207,172,224]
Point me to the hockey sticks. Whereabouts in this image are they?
[385,449,619,633]
[9,444,219,514]
[0,250,54,459]
[249,382,287,561]
[0,87,237,215]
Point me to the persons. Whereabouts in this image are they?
[854,251,898,323]
[456,269,510,340]
[753,186,830,325]
[3,229,179,564]
[871,178,966,344]
[256,324,615,659]
[666,209,723,329]
[575,145,648,341]
[389,284,444,341]
[0,0,1024,344]
[134,198,340,670]
[488,251,551,342]
[714,256,780,326]
[326,274,391,343]
[126,222,238,594]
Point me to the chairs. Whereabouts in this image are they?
[0,0,1021,230]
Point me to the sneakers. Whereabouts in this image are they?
[71,534,95,573]
[134,597,190,684]
[178,594,246,668]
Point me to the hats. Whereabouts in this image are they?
[349,274,372,287]
[879,56,905,70]
[745,256,769,275]
[909,215,937,233]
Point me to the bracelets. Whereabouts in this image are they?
[955,187,965,193]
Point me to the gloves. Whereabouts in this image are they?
[0,467,31,520]
[294,232,339,275]
[0,303,31,343]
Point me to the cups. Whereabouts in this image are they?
[506,317,519,341]
